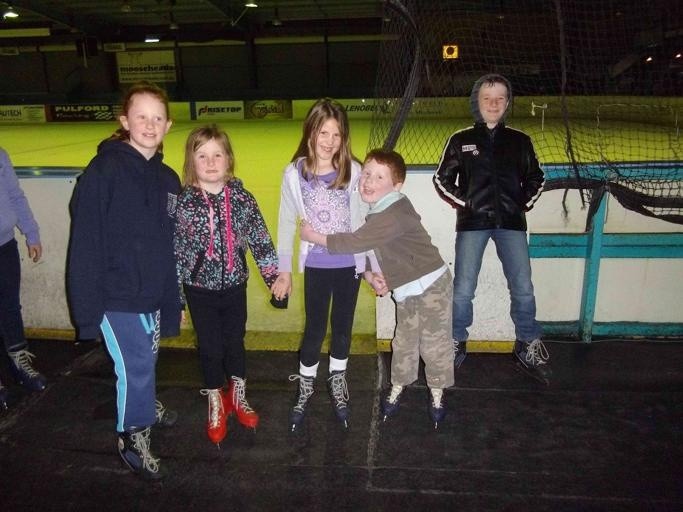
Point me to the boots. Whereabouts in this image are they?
[452,339,467,368]
[513,337,551,379]
[226,379,257,429]
[119,426,163,479]
[290,373,315,424]
[0,387,14,403]
[328,369,350,421]
[155,402,176,425]
[206,387,227,444]
[8,347,47,390]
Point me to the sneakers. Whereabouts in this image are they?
[430,387,448,422]
[382,381,406,416]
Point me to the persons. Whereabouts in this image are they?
[0,144,47,411]
[173,120,292,444]
[269,95,387,424]
[62,78,185,485]
[432,74,553,380]
[295,146,456,422]
